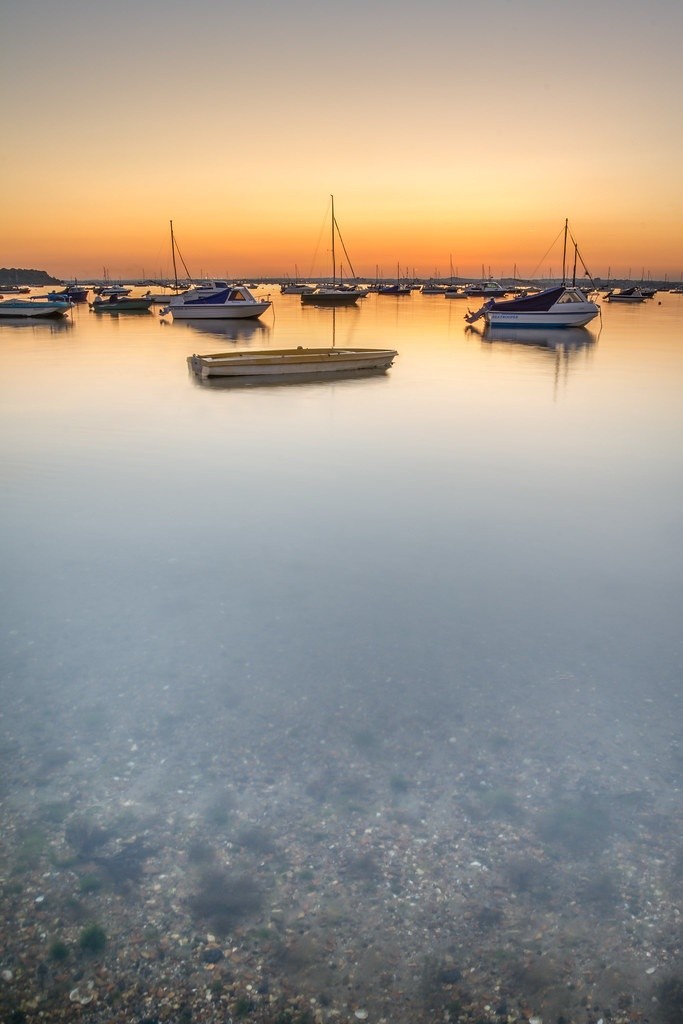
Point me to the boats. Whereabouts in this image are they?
[0,282,171,317]
[187,348,398,388]
[483,219,601,327]
[276,195,483,306]
[169,221,272,320]
[603,268,683,304]
